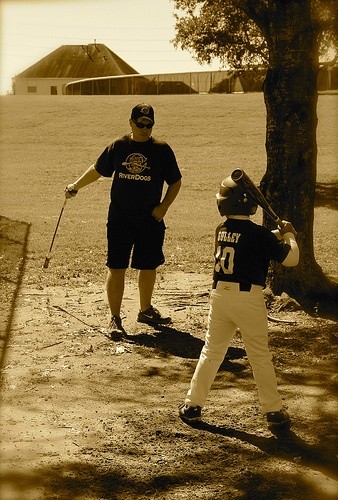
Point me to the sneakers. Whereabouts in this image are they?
[267,409,291,426]
[108,315,125,341]
[137,305,171,324]
[178,402,202,422]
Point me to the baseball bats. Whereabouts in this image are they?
[230,168,284,228]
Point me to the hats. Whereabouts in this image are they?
[130,102,155,124]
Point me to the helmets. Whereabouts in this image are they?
[216,174,259,217]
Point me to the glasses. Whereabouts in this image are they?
[132,118,153,129]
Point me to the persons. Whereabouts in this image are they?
[64,103,182,339]
[178,174,300,429]
